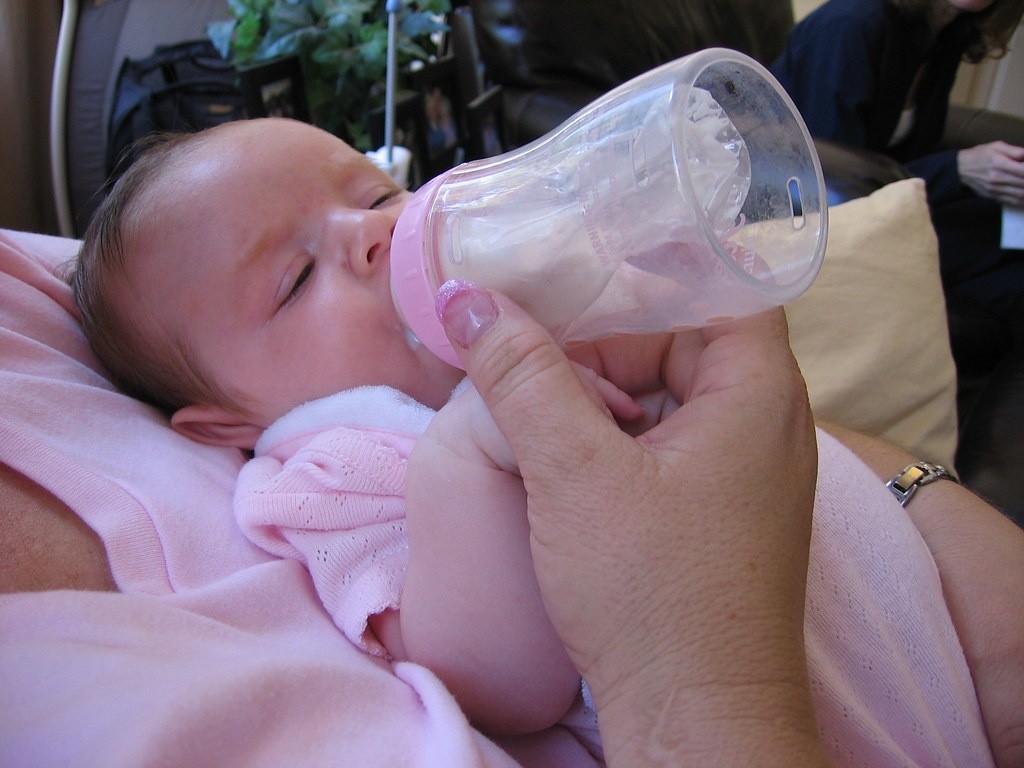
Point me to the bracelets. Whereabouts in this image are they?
[885,457,959,512]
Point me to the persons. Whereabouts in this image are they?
[73,115,990,766]
[766,0,1024,444]
[1,227,1024,767]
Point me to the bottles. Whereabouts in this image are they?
[385,47,830,375]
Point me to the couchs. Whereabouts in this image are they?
[454,0,1024,436]
